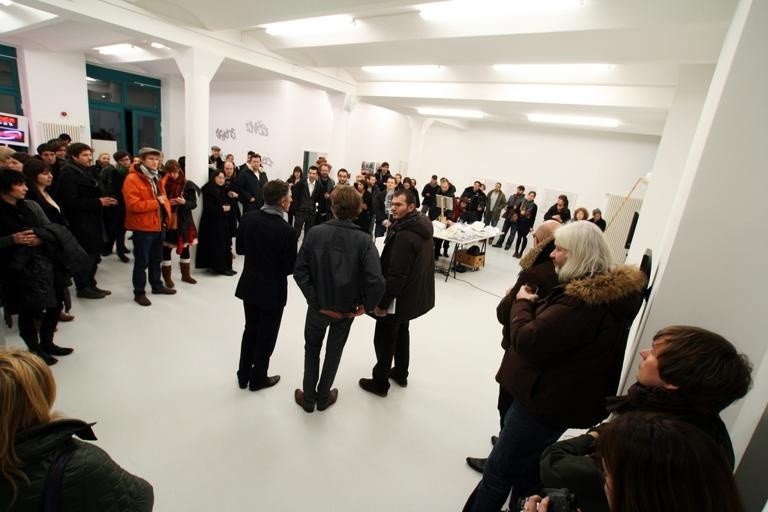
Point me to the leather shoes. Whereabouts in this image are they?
[359,369,406,396]
[135,295,150,305]
[152,285,176,294]
[60,311,73,321]
[36,343,73,364]
[77,286,112,299]
[240,375,280,390]
[296,389,338,412]
[467,436,499,472]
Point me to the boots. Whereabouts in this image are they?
[162,261,173,288]
[180,259,197,284]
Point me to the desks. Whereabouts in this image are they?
[432,229,506,281]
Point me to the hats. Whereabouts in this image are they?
[139,147,160,156]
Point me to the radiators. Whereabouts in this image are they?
[38,119,81,146]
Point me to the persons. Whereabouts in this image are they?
[462,219,647,512]
[234,179,298,390]
[466,220,561,474]
[0,346,153,512]
[360,189,435,397]
[540,326,753,496]
[522,412,743,512]
[293,185,386,413]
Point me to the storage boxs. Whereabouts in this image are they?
[457,249,484,267]
